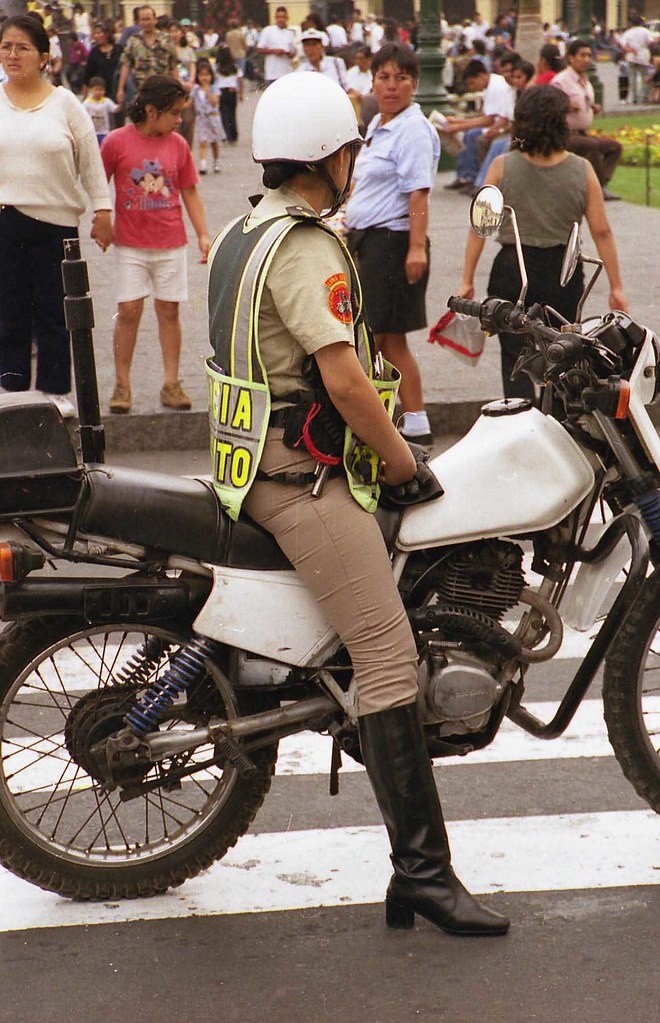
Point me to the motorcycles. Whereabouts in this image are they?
[0,186,660,900]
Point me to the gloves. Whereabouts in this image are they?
[381,443,444,506]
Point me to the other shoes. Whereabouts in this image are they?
[602,188,620,201]
[214,162,220,172]
[221,139,237,148]
[400,428,435,449]
[444,180,473,190]
[199,165,207,174]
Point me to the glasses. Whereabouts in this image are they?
[0,44,39,58]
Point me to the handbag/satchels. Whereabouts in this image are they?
[428,307,485,368]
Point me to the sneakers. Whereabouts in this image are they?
[46,394,76,418]
[110,384,131,414]
[160,382,191,410]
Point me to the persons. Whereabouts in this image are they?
[203,70,511,939]
[459,83,627,398]
[90,74,211,410]
[0,13,115,417]
[341,42,442,446]
[0,0,660,202]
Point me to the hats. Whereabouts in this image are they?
[301,28,322,44]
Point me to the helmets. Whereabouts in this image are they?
[251,71,366,163]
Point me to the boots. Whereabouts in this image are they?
[355,700,508,937]
